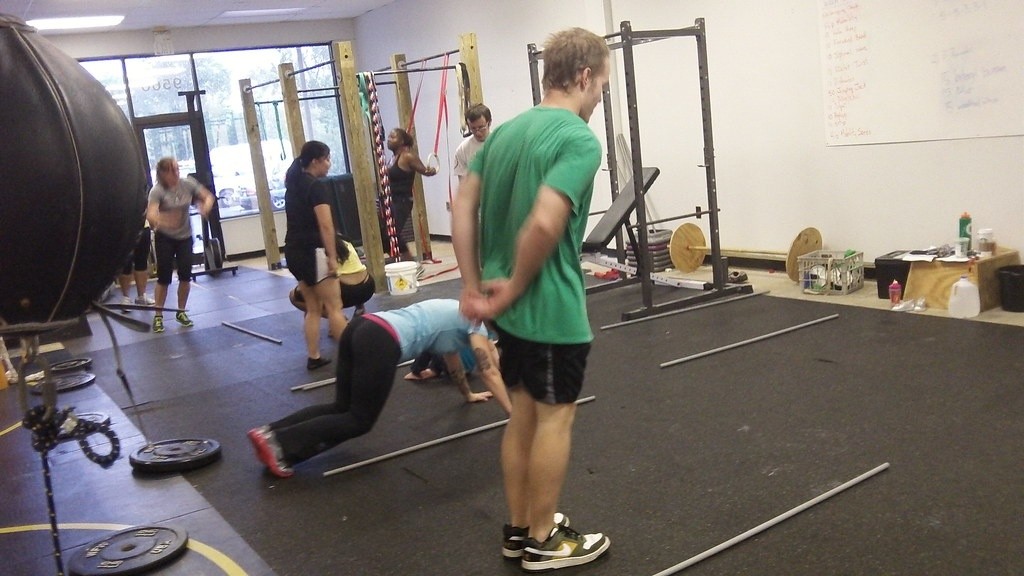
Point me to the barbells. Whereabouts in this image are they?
[669,221,824,284]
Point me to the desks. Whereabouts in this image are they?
[905,248,1018,312]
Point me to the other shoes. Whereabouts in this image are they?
[120,296,132,311]
[135,293,155,305]
[306,356,332,370]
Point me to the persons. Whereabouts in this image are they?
[119,219,156,312]
[378,128,436,260]
[289,223,375,335]
[451,27,610,570]
[146,157,214,332]
[248,297,511,477]
[285,140,347,370]
[454,103,492,178]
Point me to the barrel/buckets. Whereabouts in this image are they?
[384,261,419,296]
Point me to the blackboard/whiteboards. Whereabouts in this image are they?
[817,0,1024,147]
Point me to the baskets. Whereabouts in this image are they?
[797,250,863,296]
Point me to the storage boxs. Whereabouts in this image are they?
[874,250,911,300]
[797,249,864,296]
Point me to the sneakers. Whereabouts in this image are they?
[176,311,194,326]
[152,315,165,332]
[256,430,295,477]
[246,425,271,455]
[502,512,570,557]
[522,523,611,571]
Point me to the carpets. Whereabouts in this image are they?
[71,263,1023,576]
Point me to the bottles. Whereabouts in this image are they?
[889,279,901,308]
[948,275,979,319]
[959,212,972,250]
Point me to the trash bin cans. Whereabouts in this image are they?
[999,265,1024,313]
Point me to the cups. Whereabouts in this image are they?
[976,229,995,257]
[956,237,969,257]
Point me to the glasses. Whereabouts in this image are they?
[468,120,489,134]
